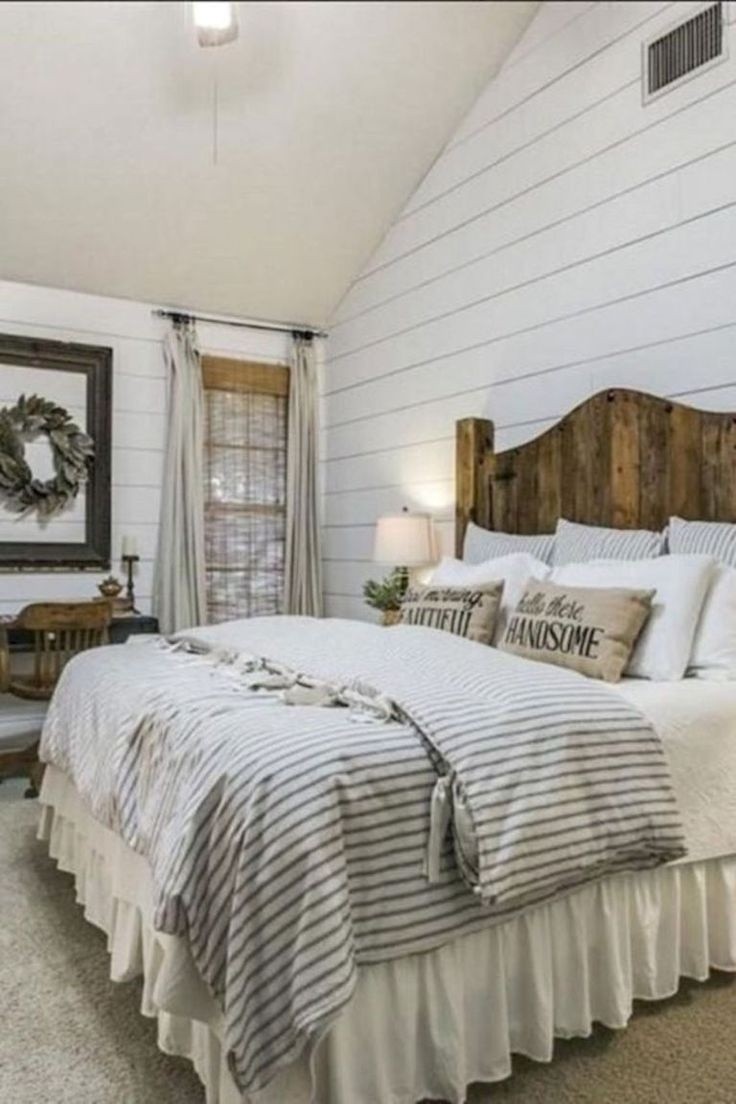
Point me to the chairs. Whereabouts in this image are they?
[8,599,114,701]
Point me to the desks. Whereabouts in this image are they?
[0,616,159,656]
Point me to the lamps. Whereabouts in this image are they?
[371,516,430,594]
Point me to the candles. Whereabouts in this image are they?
[121,534,136,555]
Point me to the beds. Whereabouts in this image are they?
[35,389,736,1104]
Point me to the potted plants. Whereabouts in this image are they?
[362,567,408,626]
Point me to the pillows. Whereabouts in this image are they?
[400,579,505,646]
[668,516,736,568]
[551,517,666,567]
[498,575,658,682]
[463,521,556,565]
[550,555,717,683]
[430,553,549,646]
